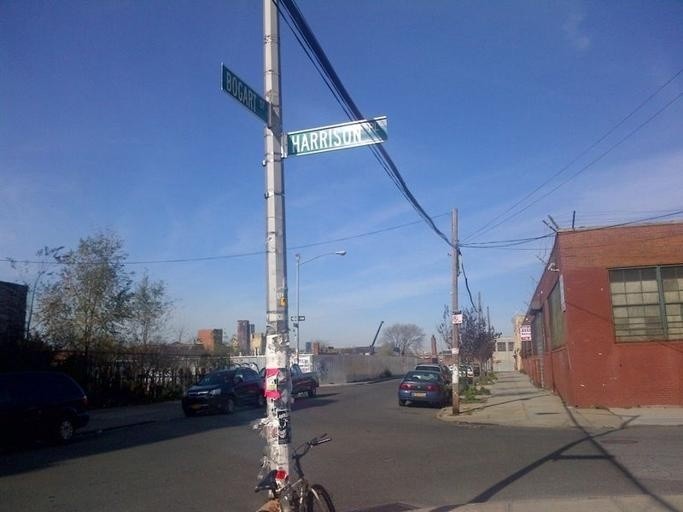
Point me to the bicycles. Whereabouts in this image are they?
[254,433,334,511]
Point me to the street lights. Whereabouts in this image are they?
[290,247,347,369]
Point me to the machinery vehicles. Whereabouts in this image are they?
[363,320,384,355]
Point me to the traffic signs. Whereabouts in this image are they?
[280,115,388,155]
[220,63,270,124]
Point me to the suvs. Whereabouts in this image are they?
[412,362,451,383]
[179,366,265,416]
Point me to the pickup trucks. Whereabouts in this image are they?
[258,364,319,400]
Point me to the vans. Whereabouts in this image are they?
[0,370,90,450]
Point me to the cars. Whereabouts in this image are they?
[449,363,482,380]
[396,370,451,408]
